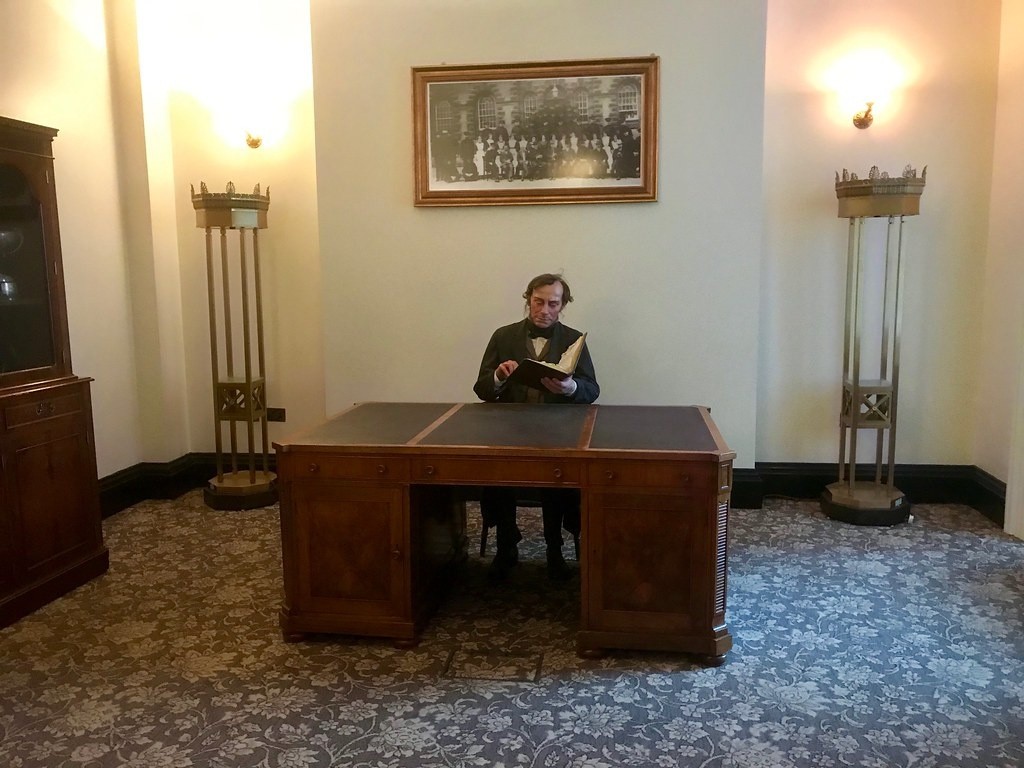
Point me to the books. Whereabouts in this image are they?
[506,331,587,392]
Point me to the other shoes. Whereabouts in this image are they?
[488,544,518,580]
[546,544,570,582]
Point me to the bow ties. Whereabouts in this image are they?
[528,328,553,339]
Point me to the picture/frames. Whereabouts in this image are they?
[411,56,656,207]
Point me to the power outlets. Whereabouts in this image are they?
[254,408,286,421]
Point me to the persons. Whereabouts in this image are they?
[473,275,600,582]
[435,115,641,180]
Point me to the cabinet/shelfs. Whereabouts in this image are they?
[0,117,109,630]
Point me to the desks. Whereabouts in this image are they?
[273,401,738,668]
